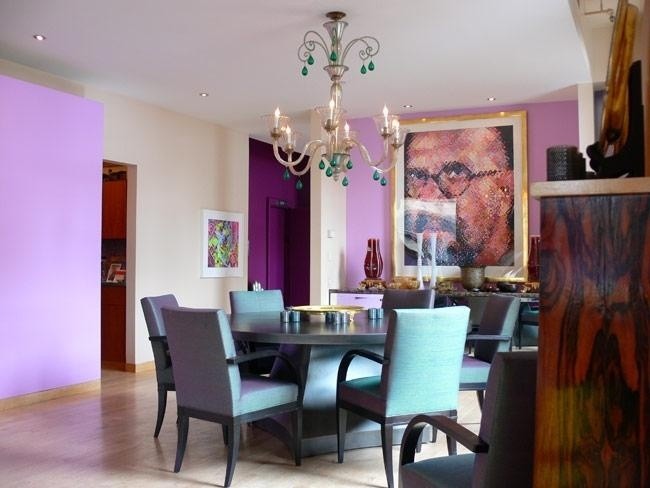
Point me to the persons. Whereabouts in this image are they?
[404,127,514,267]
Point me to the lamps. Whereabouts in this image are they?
[261,7,407,190]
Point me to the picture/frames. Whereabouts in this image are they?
[199,208,246,279]
[387,106,532,282]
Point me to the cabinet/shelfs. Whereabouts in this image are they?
[529,175,649,487]
[101,164,129,241]
[101,279,126,371]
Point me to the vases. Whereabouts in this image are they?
[459,263,489,292]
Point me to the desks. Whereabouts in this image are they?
[226,310,471,457]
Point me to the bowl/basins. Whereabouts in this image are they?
[498,284,517,292]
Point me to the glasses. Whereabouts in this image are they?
[405,160,502,198]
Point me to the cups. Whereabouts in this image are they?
[546,144,597,180]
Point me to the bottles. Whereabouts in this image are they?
[368,308,384,320]
[281,310,301,322]
[326,311,350,325]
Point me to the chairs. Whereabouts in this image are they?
[432,294,521,446]
[227,289,283,314]
[335,306,470,487]
[160,304,303,487]
[326,282,540,354]
[141,294,228,444]
